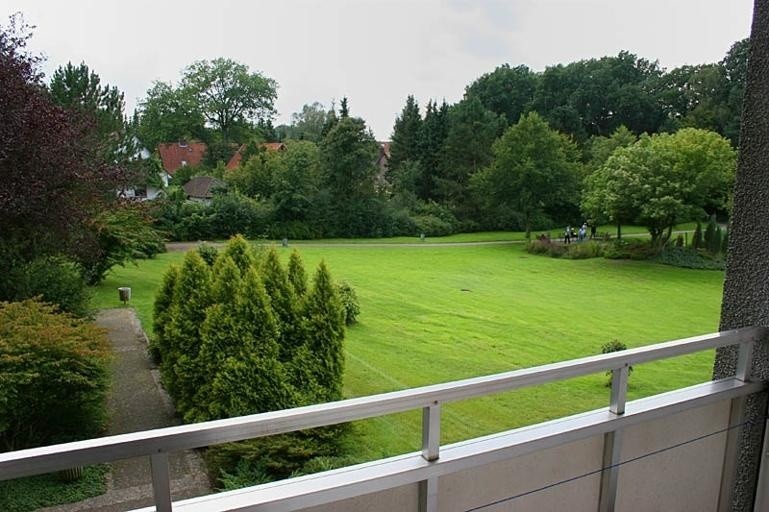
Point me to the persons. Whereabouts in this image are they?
[589,223,596,240]
[540,233,546,241]
[564,222,588,244]
